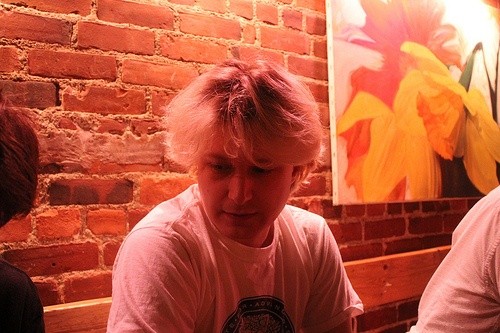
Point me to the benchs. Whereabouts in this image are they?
[42,245,453,333]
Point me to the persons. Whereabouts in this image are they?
[0,106,47,332]
[403,176,499,333]
[103,61,365,332]
